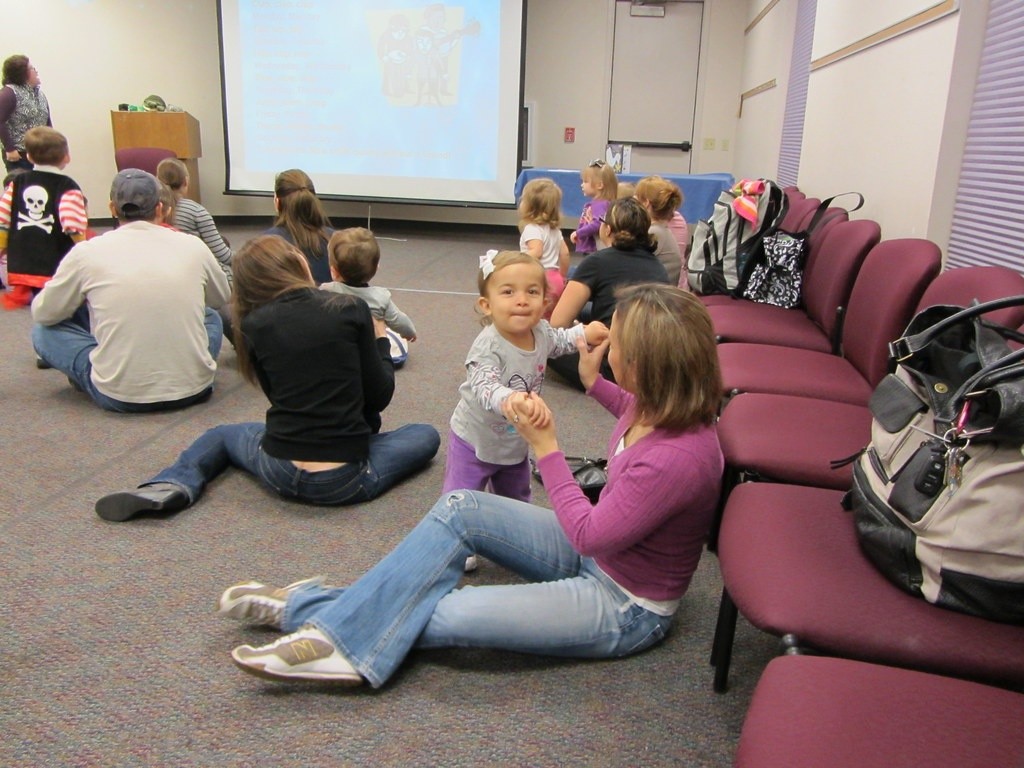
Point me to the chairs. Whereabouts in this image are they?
[688,187,1024,768]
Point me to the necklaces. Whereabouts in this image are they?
[626,424,637,449]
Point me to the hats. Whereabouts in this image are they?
[109,168,160,219]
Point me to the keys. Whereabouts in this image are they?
[942,446,968,488]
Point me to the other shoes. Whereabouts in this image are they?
[463,554,478,572]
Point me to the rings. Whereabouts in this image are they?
[512,415,519,422]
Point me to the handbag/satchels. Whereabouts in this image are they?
[830,294,1024,623]
[528,444,608,506]
[739,227,808,312]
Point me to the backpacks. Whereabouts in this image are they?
[683,177,790,299]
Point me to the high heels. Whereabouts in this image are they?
[95,483,185,523]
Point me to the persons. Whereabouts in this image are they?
[568,159,619,260]
[441,250,610,572]
[95,234,441,522]
[0,55,54,172]
[261,167,338,286]
[31,168,232,415]
[634,176,693,287]
[547,195,670,393]
[0,125,99,306]
[214,281,725,689]
[155,155,236,292]
[319,226,418,343]
[515,177,571,323]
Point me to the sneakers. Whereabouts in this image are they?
[219,577,318,633]
[231,623,364,691]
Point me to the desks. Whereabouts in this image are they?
[514,167,737,229]
